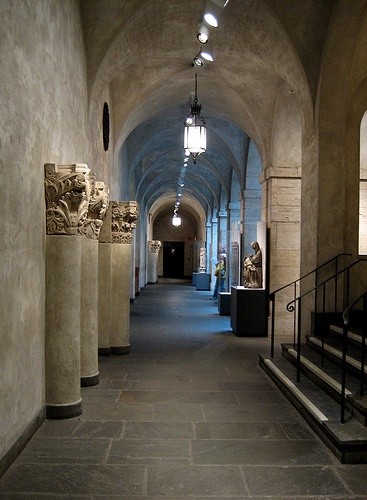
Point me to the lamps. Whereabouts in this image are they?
[183,63,207,164]
[191,0,229,73]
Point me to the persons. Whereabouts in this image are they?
[210,256,227,301]
[217,242,226,274]
[242,240,262,284]
[199,246,208,268]
